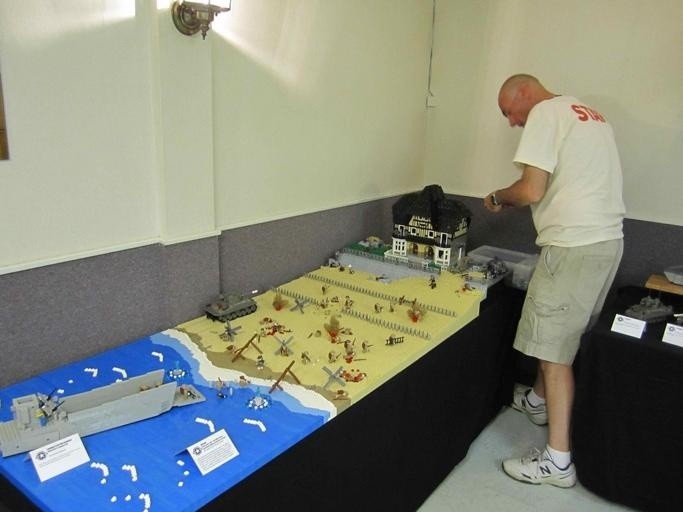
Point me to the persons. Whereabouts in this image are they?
[481,73,628,489]
[328,340,369,363]
[345,296,353,309]
[375,303,381,312]
[37,409,48,426]
[256,356,264,370]
[390,334,395,344]
[429,275,436,289]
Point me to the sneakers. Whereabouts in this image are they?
[511,387,549,426]
[502,449,577,488]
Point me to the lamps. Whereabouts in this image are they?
[172,0,232,37]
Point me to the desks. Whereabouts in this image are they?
[0,265,539,511]
[564,285,681,512]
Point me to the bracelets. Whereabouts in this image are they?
[489,192,501,208]
